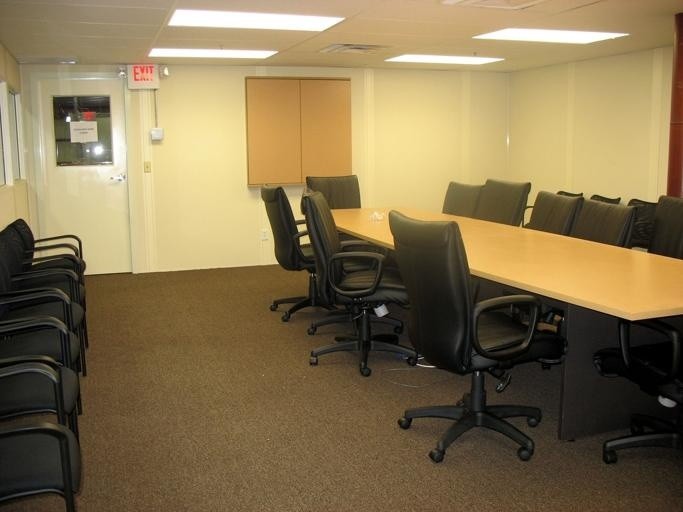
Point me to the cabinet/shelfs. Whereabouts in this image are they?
[245,76,353,187]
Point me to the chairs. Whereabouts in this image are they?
[0,218,87,511]
